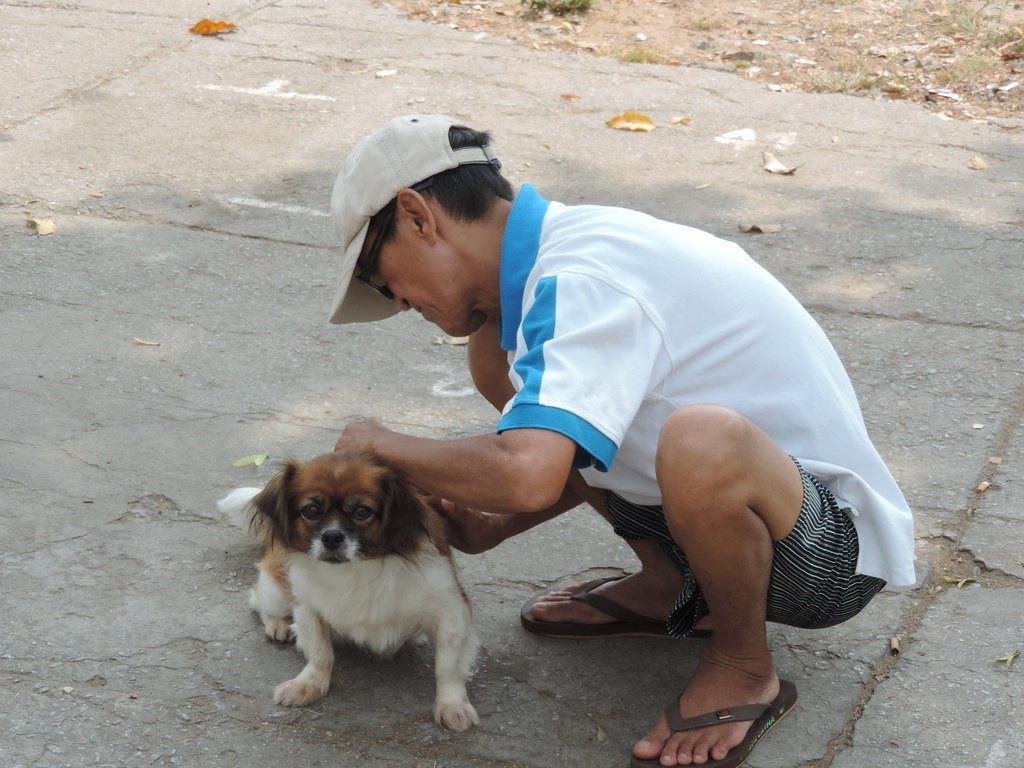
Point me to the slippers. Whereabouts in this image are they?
[520,576,717,640]
[628,676,800,768]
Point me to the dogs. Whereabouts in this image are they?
[217,444,483,735]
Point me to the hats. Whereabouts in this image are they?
[326,113,502,324]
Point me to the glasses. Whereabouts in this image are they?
[357,178,434,300]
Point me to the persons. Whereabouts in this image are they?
[328,113,917,768]
